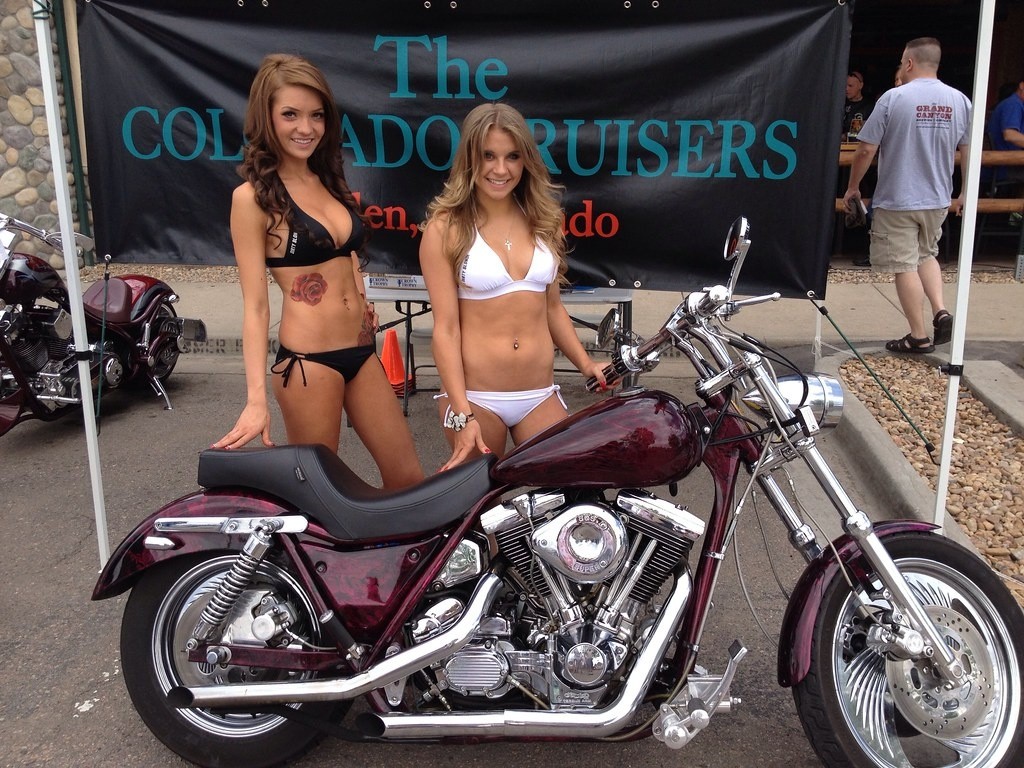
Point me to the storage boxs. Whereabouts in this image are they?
[370,271,428,290]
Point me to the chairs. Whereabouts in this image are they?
[401,302,440,417]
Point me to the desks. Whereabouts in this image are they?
[346,276,635,427]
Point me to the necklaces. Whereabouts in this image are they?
[480,206,516,251]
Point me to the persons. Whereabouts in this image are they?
[211,54,425,494]
[419,103,624,473]
[842,63,1024,266]
[844,38,973,357]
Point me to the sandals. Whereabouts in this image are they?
[932,309,954,346]
[885,333,936,354]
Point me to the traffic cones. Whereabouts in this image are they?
[381,329,414,397]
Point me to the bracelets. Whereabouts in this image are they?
[446,411,474,431]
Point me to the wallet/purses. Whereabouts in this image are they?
[844,196,867,229]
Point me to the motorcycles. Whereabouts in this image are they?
[0,214,207,443]
[91,213,1024,768]
